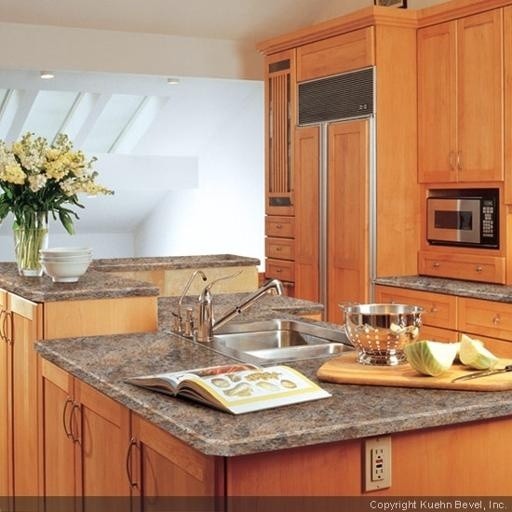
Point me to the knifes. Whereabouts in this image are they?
[451,364,512,383]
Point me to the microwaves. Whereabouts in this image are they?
[426,195,500,249]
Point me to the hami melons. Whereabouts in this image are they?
[458,332,500,371]
[453,338,484,356]
[403,340,460,378]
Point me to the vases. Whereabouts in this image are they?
[14,230,48,277]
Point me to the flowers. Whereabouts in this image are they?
[0,130,116,270]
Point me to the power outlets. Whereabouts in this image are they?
[365,437,392,492]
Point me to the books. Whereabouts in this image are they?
[124,365,332,415]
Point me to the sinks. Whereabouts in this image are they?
[221,342,356,365]
[165,318,344,352]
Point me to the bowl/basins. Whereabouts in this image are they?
[39,247,96,283]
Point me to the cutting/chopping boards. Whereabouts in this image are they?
[316,352,512,391]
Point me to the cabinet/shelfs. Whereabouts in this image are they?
[41,356,133,512]
[263,47,293,295]
[420,248,511,287]
[376,285,511,360]
[414,9,504,183]
[501,6,511,205]
[1,288,45,512]
[134,413,227,511]
[294,27,372,329]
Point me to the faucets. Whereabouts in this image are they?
[177,269,206,312]
[212,277,284,337]
